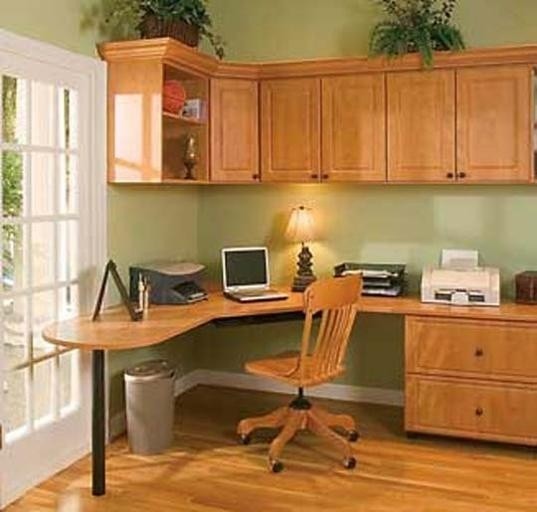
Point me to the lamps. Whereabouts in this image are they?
[281,206,325,295]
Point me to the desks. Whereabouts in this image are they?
[41,285,536,496]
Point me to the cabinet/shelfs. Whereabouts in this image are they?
[260,43,537,186]
[104,39,259,187]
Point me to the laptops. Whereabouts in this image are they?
[221,246,288,302]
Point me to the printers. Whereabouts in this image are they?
[129,258,208,306]
[417,247,502,307]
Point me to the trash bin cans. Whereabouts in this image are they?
[124,358,177,454]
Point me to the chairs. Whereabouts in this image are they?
[232,272,365,473]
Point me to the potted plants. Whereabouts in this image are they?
[364,2,468,67]
[98,0,230,61]
